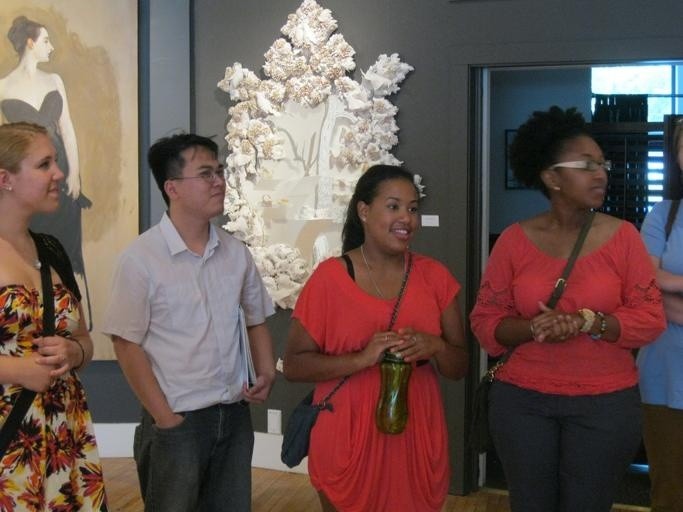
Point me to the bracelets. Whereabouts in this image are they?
[68,336,85,370]
[578,307,597,334]
[591,311,608,340]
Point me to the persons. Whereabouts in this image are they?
[101,131,279,512]
[0,120,110,512]
[0,14,93,334]
[635,117,683,511]
[466,105,670,512]
[282,161,473,512]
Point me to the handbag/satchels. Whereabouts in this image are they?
[466,378,494,456]
[279,392,325,469]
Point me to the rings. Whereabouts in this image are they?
[386,335,390,341]
[412,336,417,341]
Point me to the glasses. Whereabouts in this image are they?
[160,167,226,184]
[547,157,615,172]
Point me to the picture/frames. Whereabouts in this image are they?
[0,0,150,369]
[504,128,538,190]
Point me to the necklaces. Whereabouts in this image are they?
[15,230,42,271]
[359,242,405,300]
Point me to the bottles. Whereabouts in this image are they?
[376,347,412,435]
